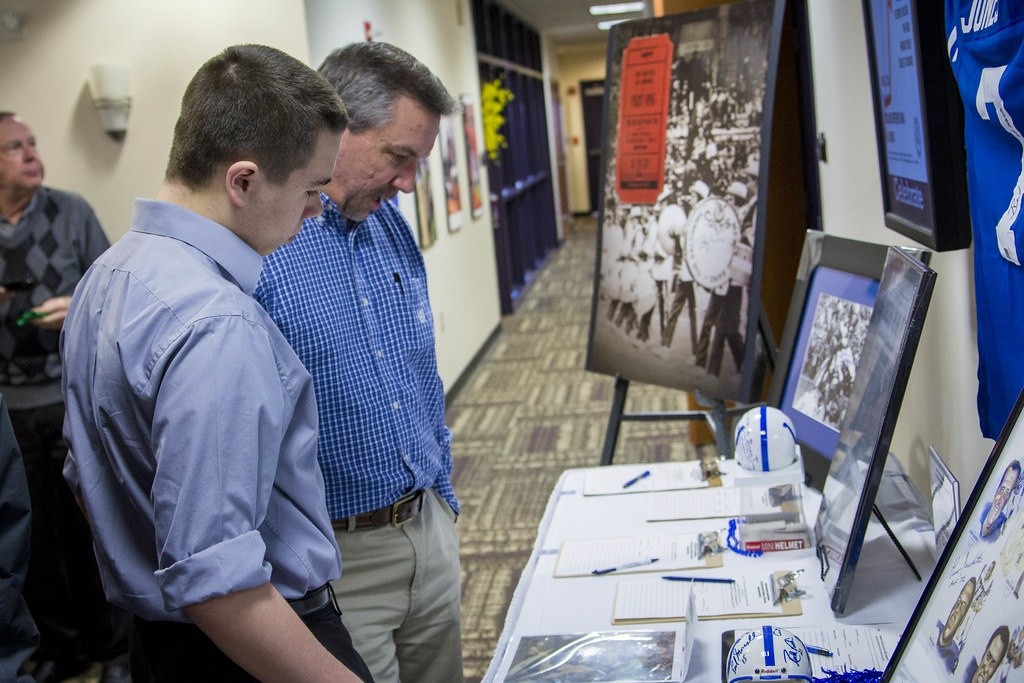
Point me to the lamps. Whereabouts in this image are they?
[86,61,132,140]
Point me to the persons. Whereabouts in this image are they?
[0,109,131,683]
[599,48,765,378]
[0,394,41,683]
[801,296,872,429]
[254,39,464,683]
[60,43,375,683]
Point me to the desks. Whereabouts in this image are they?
[481,459,937,683]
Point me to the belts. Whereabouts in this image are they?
[331,488,423,530]
[288,584,332,617]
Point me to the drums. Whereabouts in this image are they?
[682,197,741,290]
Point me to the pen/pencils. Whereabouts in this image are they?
[623,470,650,488]
[661,576,736,585]
[592,558,659,576]
[738,513,784,523]
[806,644,833,657]
[393,271,405,297]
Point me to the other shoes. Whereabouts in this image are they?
[99,652,132,683]
[31,653,87,683]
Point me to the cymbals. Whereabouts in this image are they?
[658,204,688,253]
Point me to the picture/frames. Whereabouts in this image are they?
[876,387,1024,683]
[812,244,938,615]
[767,227,933,497]
